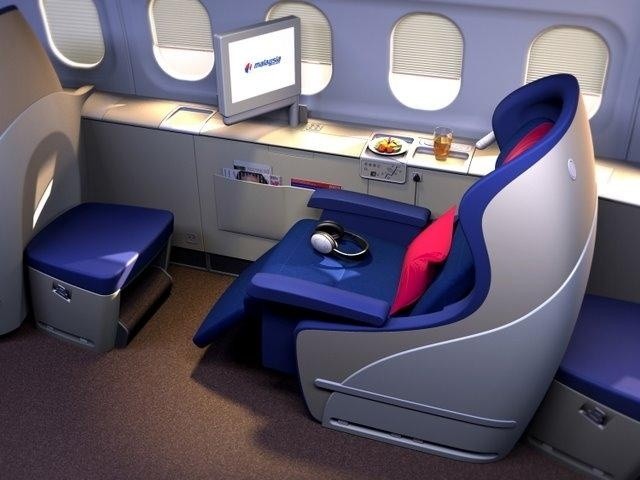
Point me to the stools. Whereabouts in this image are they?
[20,204,174,353]
[527,290,640,480]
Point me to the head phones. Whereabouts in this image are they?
[310,221,369,262]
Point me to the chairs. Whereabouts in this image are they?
[191,73,599,462]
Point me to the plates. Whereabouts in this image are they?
[368,136,410,156]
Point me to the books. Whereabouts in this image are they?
[222,159,341,192]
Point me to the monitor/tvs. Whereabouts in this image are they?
[213,15,300,125]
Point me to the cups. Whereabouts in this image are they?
[433,126,453,161]
